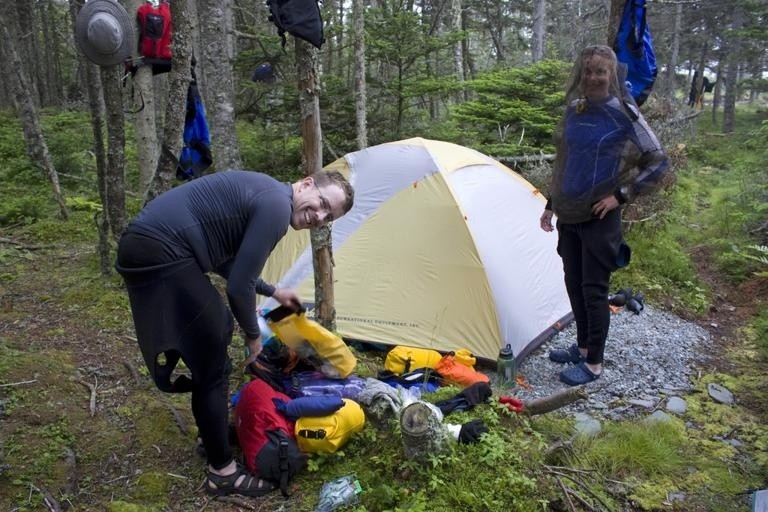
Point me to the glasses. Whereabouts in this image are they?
[315,183,334,223]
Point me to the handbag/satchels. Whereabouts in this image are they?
[231,302,368,493]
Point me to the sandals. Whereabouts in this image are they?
[204,462,281,498]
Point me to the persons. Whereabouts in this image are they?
[538,45,667,385]
[111,169,353,498]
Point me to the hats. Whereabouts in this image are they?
[75,0,135,67]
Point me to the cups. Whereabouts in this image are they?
[497,343,517,390]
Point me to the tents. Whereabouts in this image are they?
[247,136,623,379]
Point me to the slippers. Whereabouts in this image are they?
[549,343,586,363]
[559,362,601,386]
